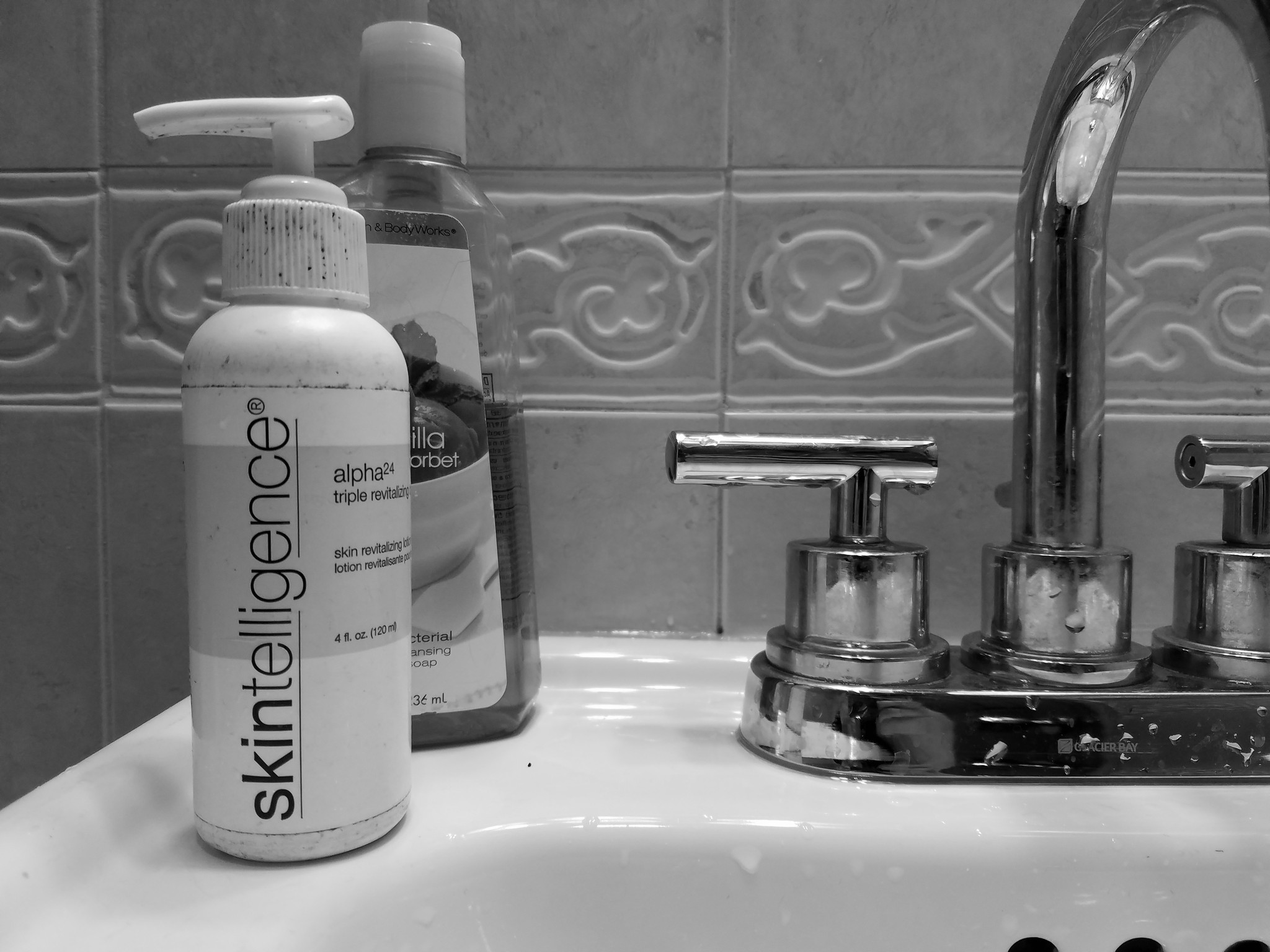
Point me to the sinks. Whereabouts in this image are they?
[5,641,1270,952]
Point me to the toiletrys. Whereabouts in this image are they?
[351,13,542,753]
[137,87,420,868]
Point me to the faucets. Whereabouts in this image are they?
[664,0,1267,791]
